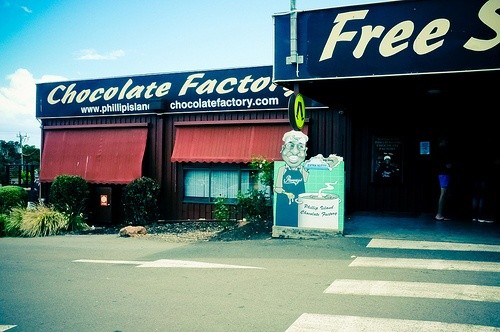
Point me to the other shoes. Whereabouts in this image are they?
[471,216,496,224]
[434,213,456,221]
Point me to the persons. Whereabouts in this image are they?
[471,194,486,222]
[486,212,498,225]
[434,137,450,220]
